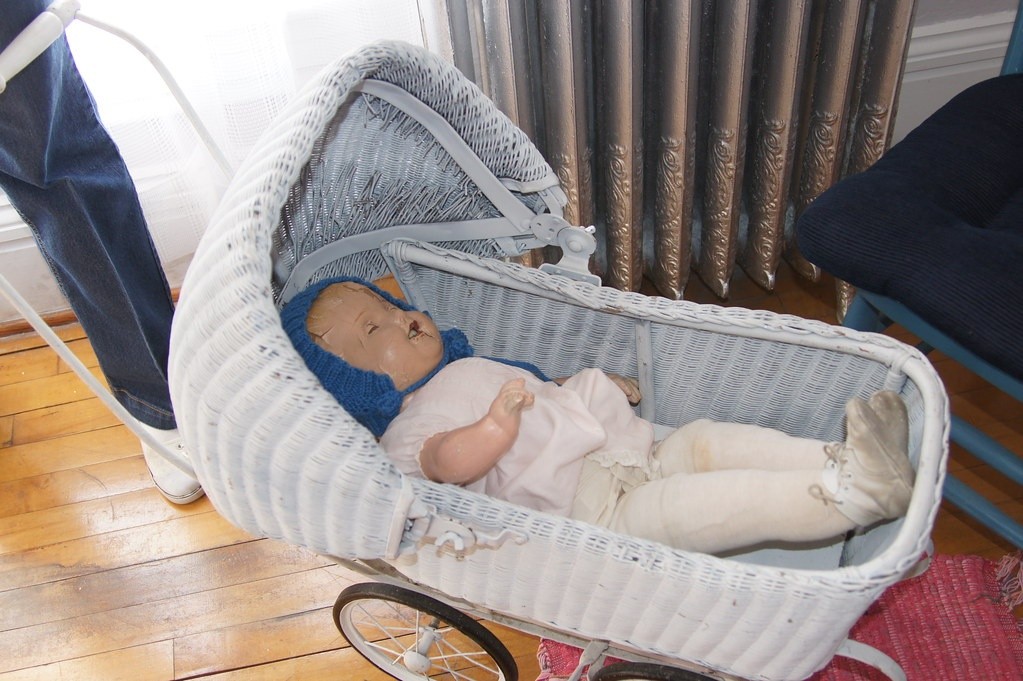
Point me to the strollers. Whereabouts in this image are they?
[0,0,950,681]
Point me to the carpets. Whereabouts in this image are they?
[536,549,1023,681]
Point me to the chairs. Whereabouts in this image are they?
[799,0,1023,551]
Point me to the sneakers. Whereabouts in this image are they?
[140,424,205,504]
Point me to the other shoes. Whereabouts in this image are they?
[808,389,916,529]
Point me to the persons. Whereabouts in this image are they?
[0,0,205,505]
[279,275,915,557]
